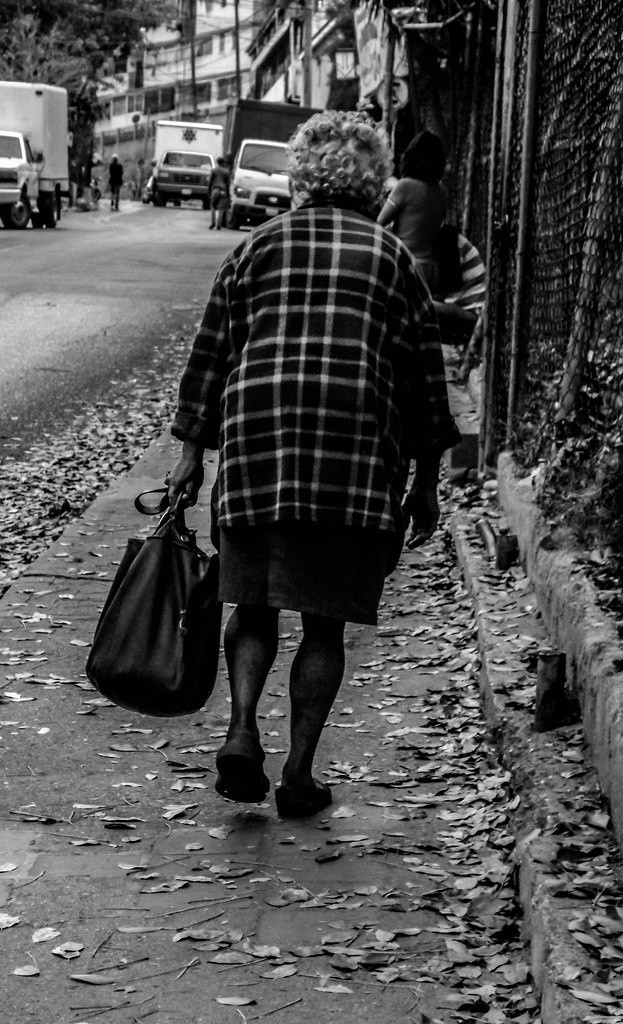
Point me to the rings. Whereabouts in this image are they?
[165,470,171,475]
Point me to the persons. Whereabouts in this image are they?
[104,153,230,232]
[375,129,485,342]
[161,110,459,819]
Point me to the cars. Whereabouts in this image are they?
[143,175,181,207]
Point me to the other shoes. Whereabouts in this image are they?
[273,778,333,821]
[215,730,271,803]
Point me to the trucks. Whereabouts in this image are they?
[0,81,70,230]
[215,99,325,231]
[150,119,223,211]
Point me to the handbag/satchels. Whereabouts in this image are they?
[85,487,221,718]
